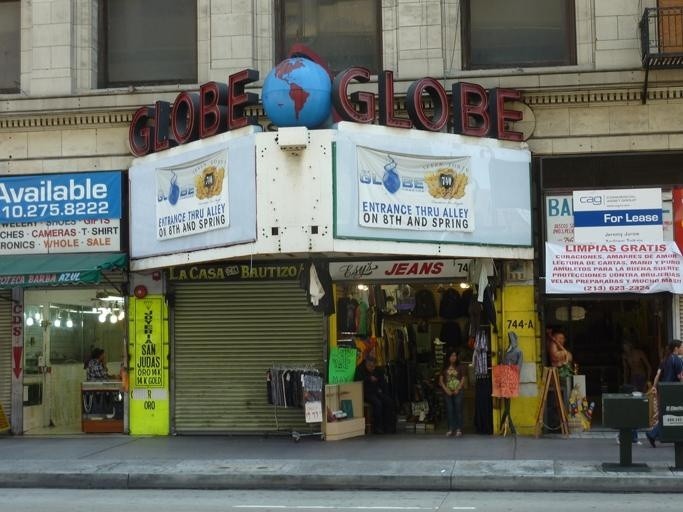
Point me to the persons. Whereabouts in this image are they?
[645,339,682,447]
[619,338,651,392]
[352,356,396,434]
[549,333,575,366]
[82,347,122,420]
[438,348,465,437]
[615,383,653,445]
[497,332,521,368]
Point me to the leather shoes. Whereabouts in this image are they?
[645,431,655,448]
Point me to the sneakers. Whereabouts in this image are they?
[455,430,461,437]
[445,431,452,436]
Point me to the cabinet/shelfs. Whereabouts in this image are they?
[321,381,366,442]
[79,380,124,433]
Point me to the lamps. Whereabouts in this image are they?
[23,288,125,328]
